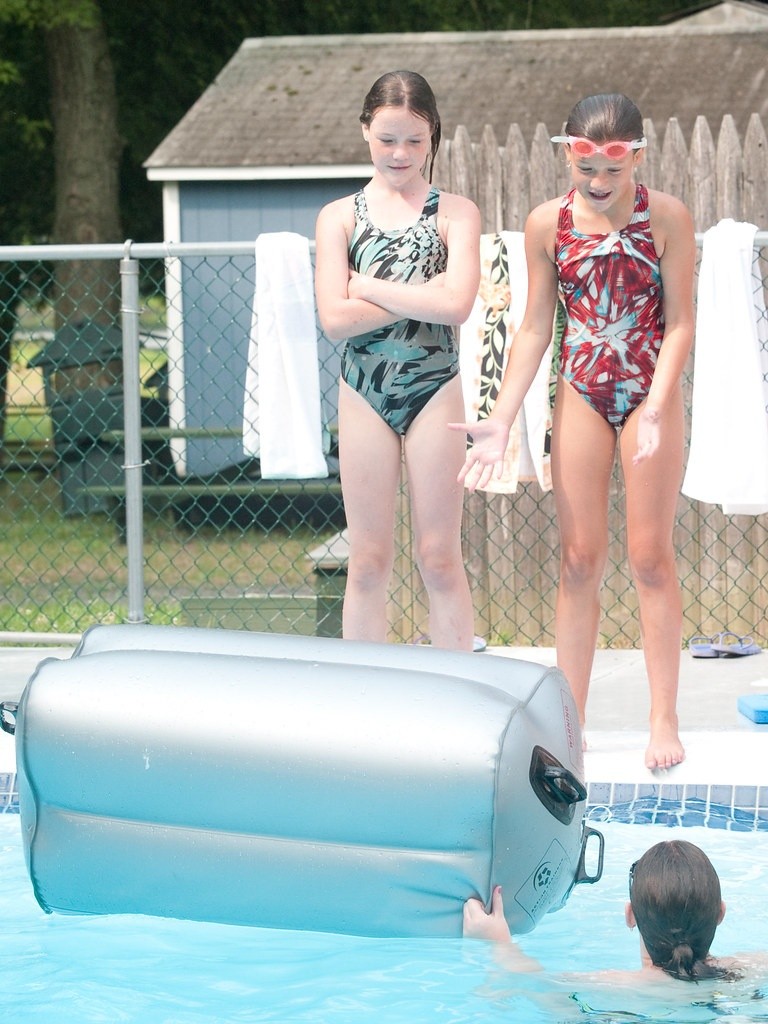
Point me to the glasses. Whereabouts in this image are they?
[570,134,634,162]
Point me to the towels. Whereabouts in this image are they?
[679,216,768,518]
[239,229,333,480]
[449,229,556,495]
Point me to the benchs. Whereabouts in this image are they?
[306,524,348,638]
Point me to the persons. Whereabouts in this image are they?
[461,839,749,999]
[448,93,694,768]
[314,69,480,646]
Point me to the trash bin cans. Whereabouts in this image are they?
[303,526,350,638]
[26,319,147,519]
